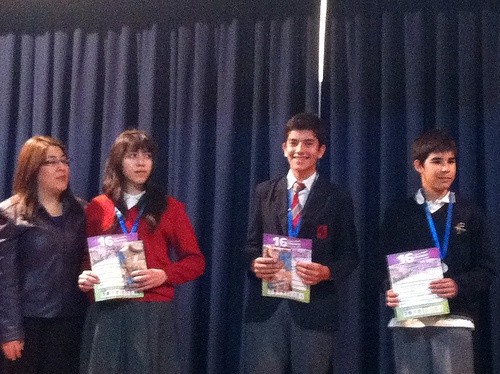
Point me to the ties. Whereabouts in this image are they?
[291,181,306,230]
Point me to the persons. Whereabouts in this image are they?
[74,128,207,374]
[241,113,359,374]
[0,135,94,374]
[375,130,496,374]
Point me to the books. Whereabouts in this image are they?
[87,233,144,301]
[385,248,450,322]
[261,233,312,302]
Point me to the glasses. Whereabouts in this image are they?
[42,157,70,166]
